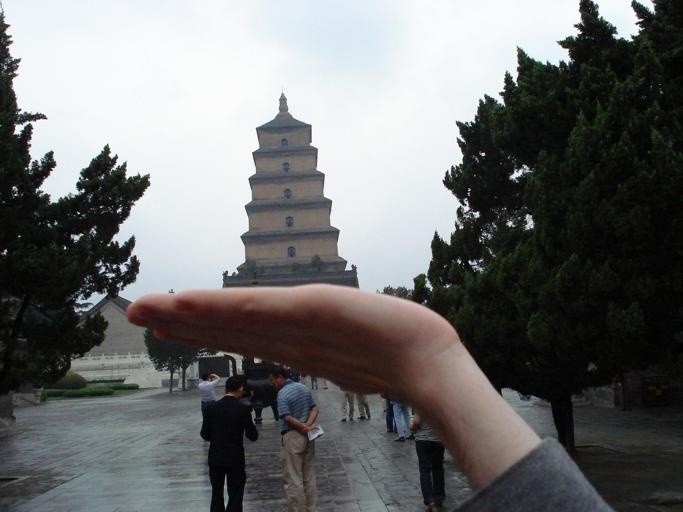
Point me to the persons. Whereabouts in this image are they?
[198,375,258,512]
[268,365,321,512]
[123,279,618,512]
[194,362,416,444]
[408,409,447,512]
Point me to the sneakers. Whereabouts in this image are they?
[425,501,443,512]
[387,429,414,441]
[341,415,370,421]
